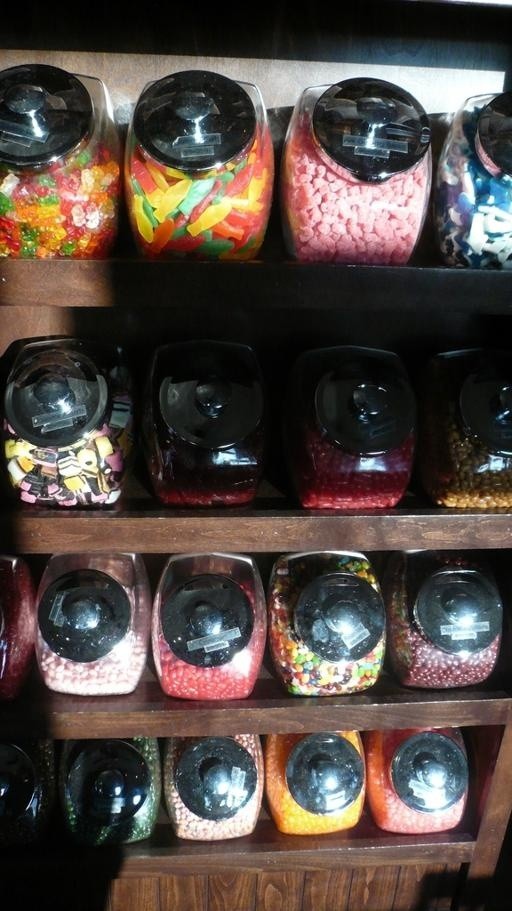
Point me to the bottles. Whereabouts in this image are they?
[417,345,511,510]
[162,733,264,841]
[379,549,503,689]
[434,85,511,270]
[1,58,123,262]
[362,725,469,834]
[1,739,57,839]
[35,553,151,697]
[139,339,274,508]
[149,552,269,702]
[277,343,416,509]
[55,736,162,847]
[3,555,36,691]
[274,75,433,268]
[267,550,387,697]
[263,730,366,836]
[123,68,276,261]
[1,333,137,510]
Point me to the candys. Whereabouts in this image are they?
[63,735,159,843]
[264,552,386,696]
[368,725,467,834]
[2,392,131,504]
[142,425,267,504]
[266,728,366,834]
[433,106,512,270]
[164,733,263,841]
[0,136,121,260]
[293,404,414,510]
[151,574,268,698]
[415,419,512,507]
[122,120,275,261]
[35,579,149,696]
[282,106,428,268]
[386,587,499,689]
[0,564,39,696]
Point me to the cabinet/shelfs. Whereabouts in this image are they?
[2,38,511,904]
